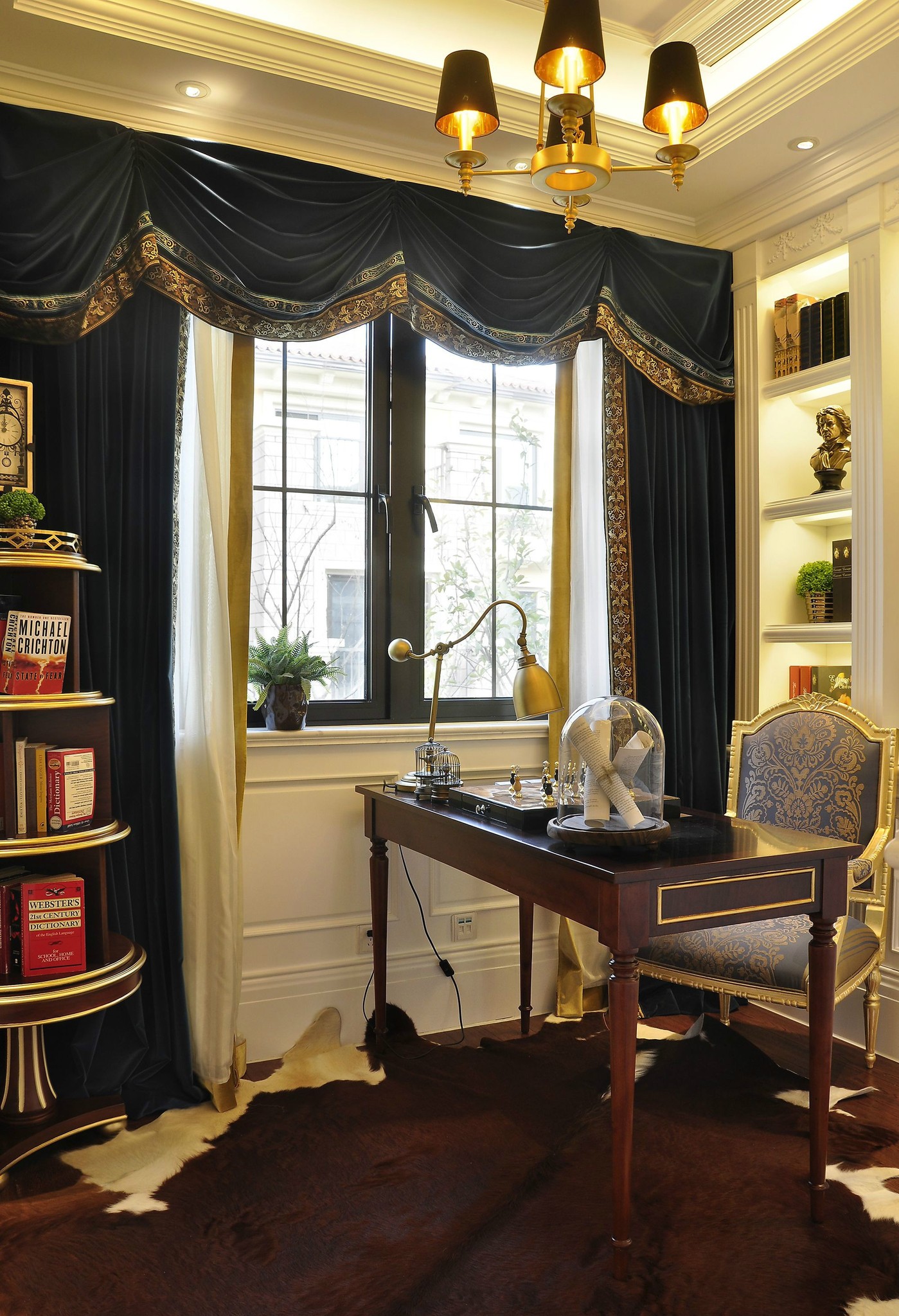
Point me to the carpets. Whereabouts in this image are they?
[1,1007,899,1316]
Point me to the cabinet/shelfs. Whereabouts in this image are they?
[0,527,151,1200]
[760,355,852,648]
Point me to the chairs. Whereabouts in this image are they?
[601,687,899,1102]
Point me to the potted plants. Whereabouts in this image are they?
[247,622,346,731]
[796,560,835,624]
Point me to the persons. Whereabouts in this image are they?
[810,404,852,472]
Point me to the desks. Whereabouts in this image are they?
[354,776,866,1284]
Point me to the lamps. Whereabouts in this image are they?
[433,1,710,236]
[387,599,565,792]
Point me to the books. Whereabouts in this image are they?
[800,305,812,371]
[811,666,851,707]
[0,865,87,977]
[775,298,787,378]
[14,736,96,834]
[835,292,850,360]
[799,665,827,695]
[842,539,852,623]
[786,293,825,374]
[0,610,71,695]
[833,539,843,622]
[811,301,824,367]
[824,297,836,364]
[789,666,800,699]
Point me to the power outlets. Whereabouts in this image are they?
[356,924,373,954]
